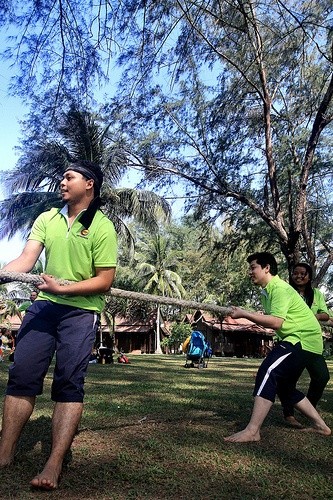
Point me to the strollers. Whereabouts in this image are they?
[185,330,213,369]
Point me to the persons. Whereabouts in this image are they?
[0,161,119,489]
[277,264,330,426]
[0,328,15,362]
[223,252,331,442]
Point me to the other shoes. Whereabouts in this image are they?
[285,421,305,429]
